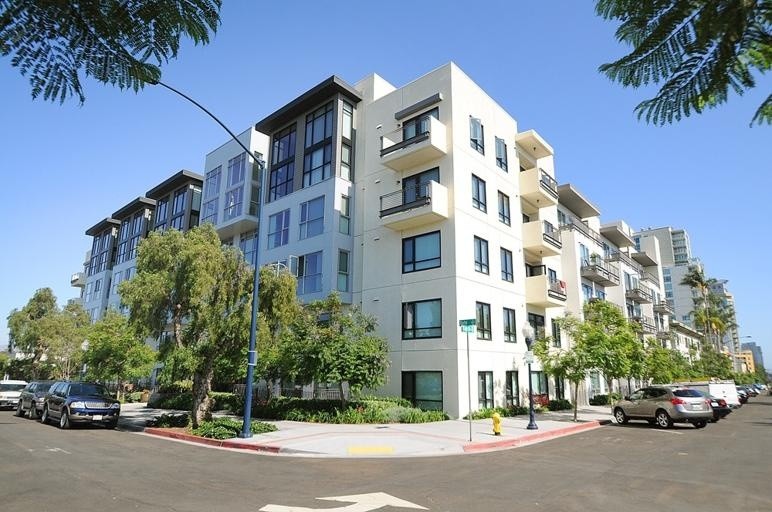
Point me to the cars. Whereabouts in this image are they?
[612,380,772,430]
[0,378,27,407]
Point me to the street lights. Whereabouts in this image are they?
[723,334,752,345]
[520,320,539,430]
[129,66,265,437]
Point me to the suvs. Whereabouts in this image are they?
[16,380,60,419]
[40,380,123,430]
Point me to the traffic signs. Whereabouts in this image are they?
[461,326,474,332]
[459,318,476,326]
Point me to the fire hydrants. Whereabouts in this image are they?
[492,410,501,436]
